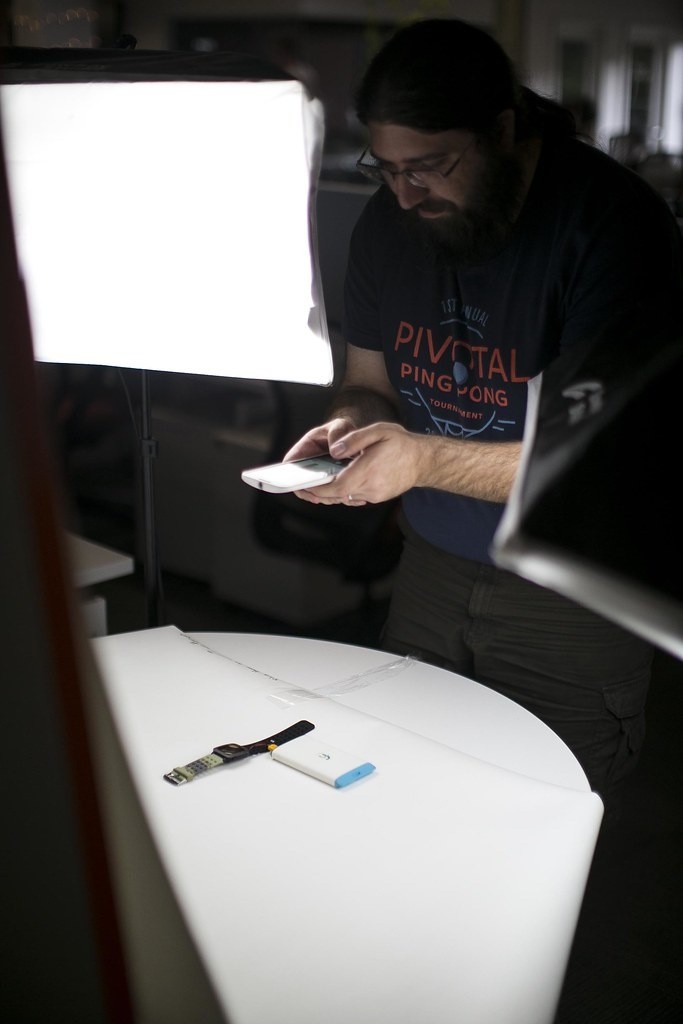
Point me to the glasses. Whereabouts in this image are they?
[355,132,479,191]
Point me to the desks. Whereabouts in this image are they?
[88,632,593,1024]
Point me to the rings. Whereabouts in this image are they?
[347,494,354,503]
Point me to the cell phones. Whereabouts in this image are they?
[241,453,356,494]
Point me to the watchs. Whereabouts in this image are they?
[162,719,315,786]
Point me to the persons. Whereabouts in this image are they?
[278,18,683,801]
